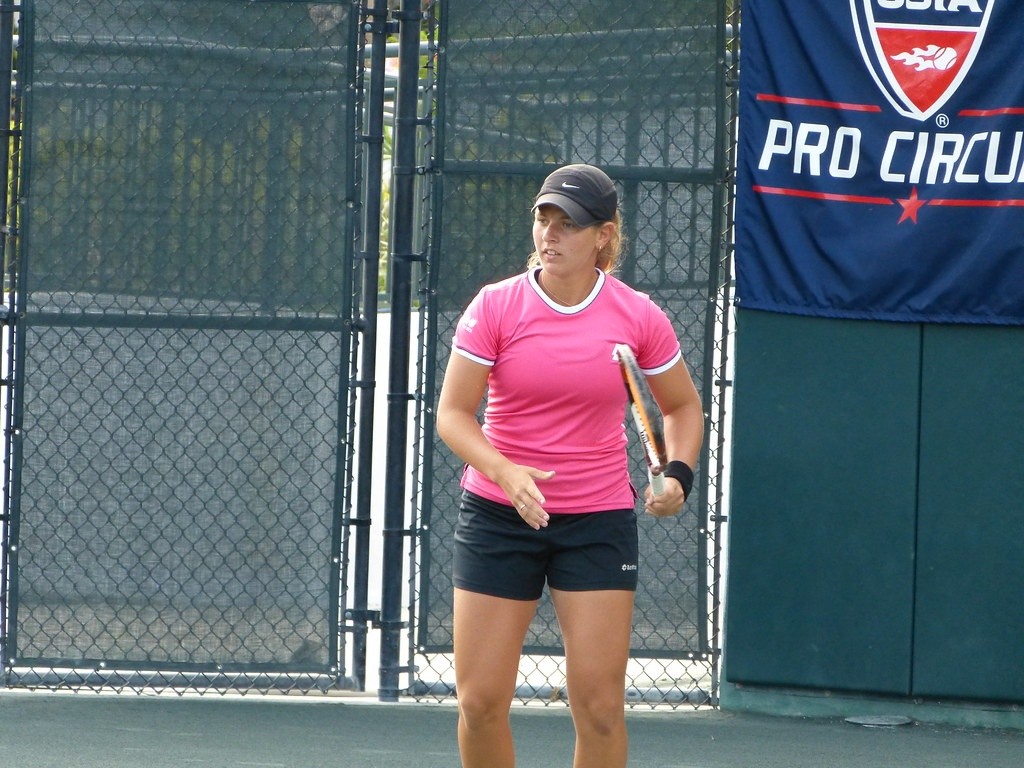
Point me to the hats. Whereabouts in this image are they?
[530,163,618,227]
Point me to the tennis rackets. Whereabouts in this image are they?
[616,344,666,497]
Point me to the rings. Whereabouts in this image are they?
[520,504,526,511]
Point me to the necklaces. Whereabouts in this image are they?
[539,270,574,307]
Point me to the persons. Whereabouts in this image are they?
[434,163,705,768]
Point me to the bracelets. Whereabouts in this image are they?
[663,461,695,501]
